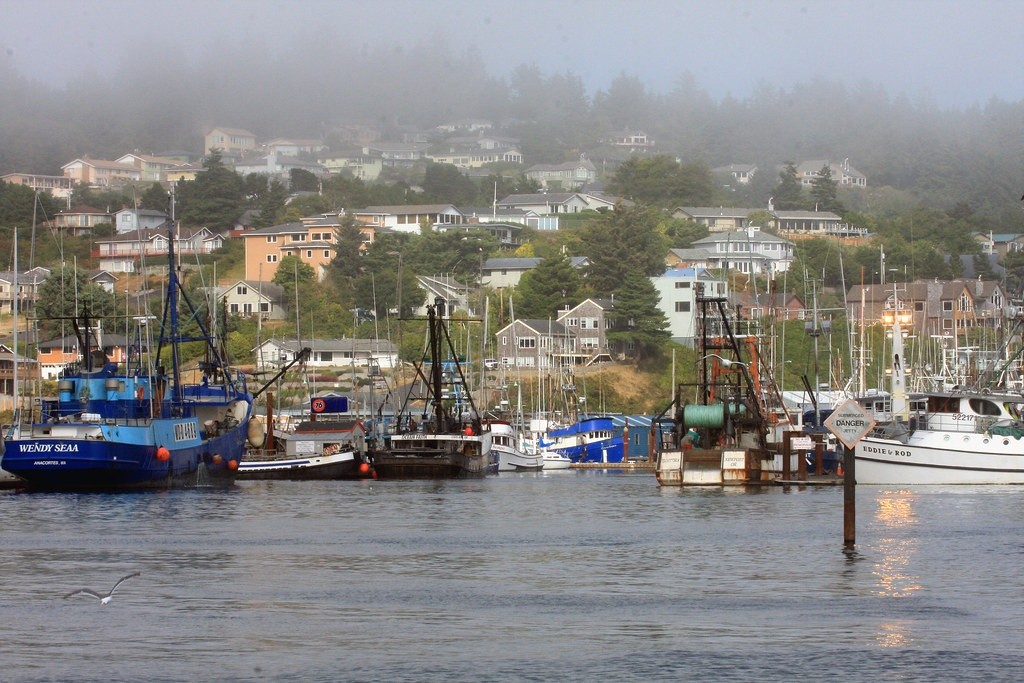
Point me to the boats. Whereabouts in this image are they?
[790,220,1024,486]
[368,299,493,480]
[463,293,547,473]
[222,251,367,479]
[2,179,254,493]
[540,294,627,464]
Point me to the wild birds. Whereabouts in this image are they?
[695,354,747,368]
[62,573,141,605]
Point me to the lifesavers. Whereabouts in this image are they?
[137,387,144,398]
[719,435,731,447]
